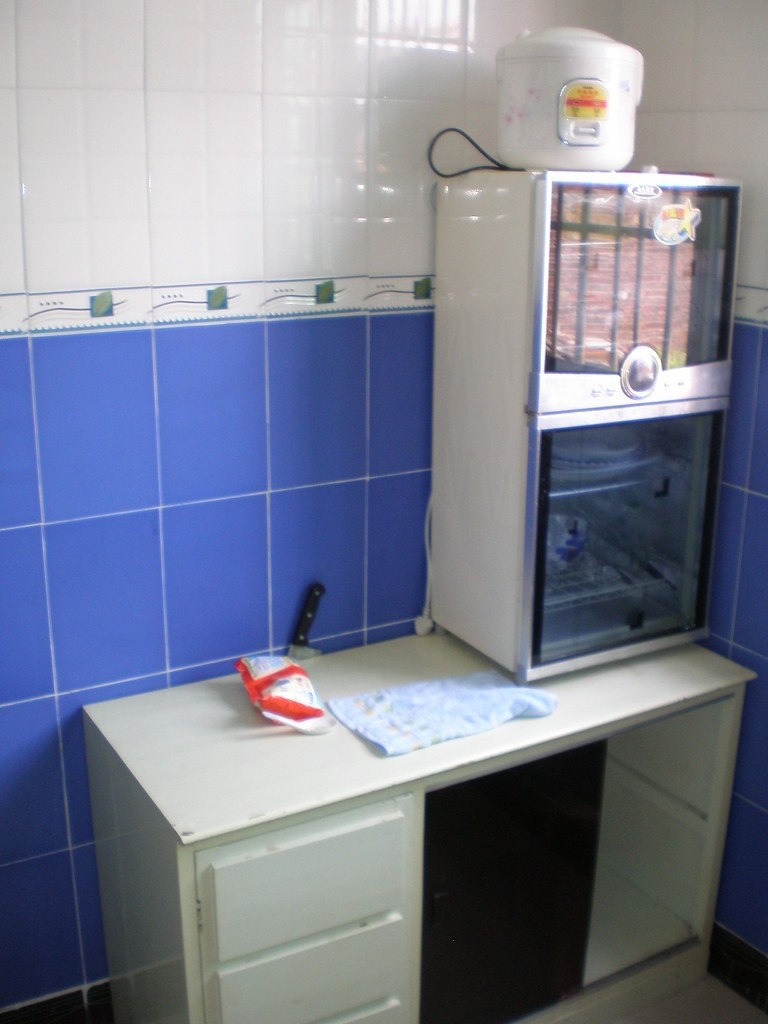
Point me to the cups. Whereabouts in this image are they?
[547,516,588,571]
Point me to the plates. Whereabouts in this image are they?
[550,428,669,483]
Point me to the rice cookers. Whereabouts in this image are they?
[498,27,644,173]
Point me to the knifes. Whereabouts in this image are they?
[287,583,325,660]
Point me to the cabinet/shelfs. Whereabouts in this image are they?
[84,634,757,1024]
